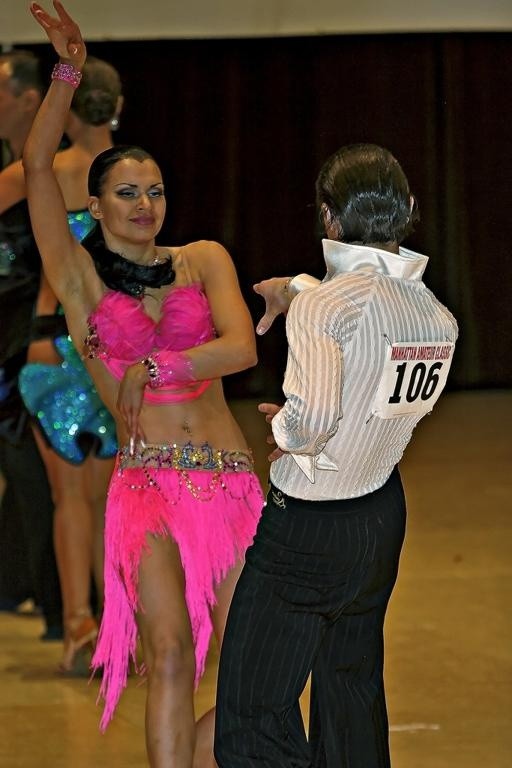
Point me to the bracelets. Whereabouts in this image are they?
[51,63,83,87]
[285,276,292,296]
[141,350,197,388]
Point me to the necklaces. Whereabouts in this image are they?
[151,248,160,265]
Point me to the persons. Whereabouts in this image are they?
[0,49,98,642]
[213,142,460,768]
[0,57,126,675]
[22,0,267,768]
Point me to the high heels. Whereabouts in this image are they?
[58,616,99,672]
